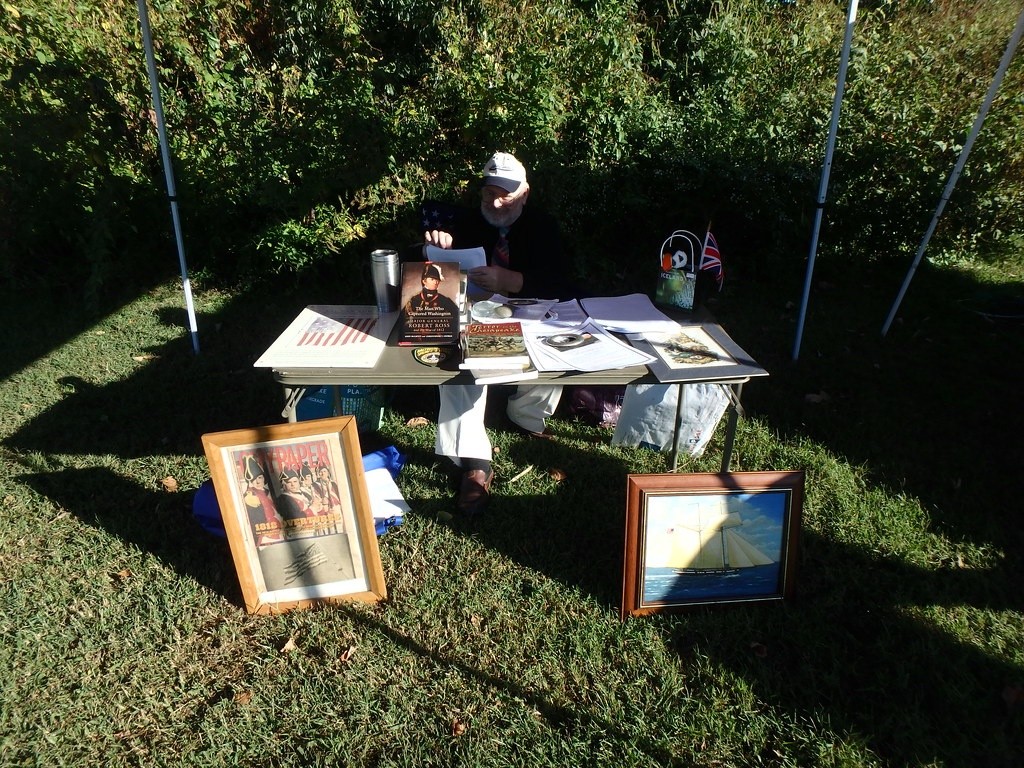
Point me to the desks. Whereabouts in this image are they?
[273,299,751,472]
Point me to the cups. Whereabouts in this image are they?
[372,249,400,313]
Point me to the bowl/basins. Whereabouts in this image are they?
[193,477,226,538]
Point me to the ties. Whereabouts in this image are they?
[491,226,511,298]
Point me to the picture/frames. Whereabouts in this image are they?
[621,470,805,624]
[201,414,387,615]
[624,316,769,383]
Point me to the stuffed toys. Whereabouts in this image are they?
[663,246,691,271]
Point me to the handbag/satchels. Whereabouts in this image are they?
[655,229,703,313]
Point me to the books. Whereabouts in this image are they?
[398,261,472,346]
[456,322,538,386]
[624,319,770,384]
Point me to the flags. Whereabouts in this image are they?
[699,232,724,293]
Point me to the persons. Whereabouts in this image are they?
[425,152,564,507]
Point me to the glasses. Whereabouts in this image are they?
[482,188,517,203]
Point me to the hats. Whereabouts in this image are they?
[421,266,441,280]
[298,461,313,482]
[481,152,527,195]
[278,462,299,488]
[315,460,331,476]
[242,454,264,487]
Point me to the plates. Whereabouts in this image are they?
[414,346,453,368]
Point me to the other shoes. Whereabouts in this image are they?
[505,414,556,439]
[455,458,494,514]
